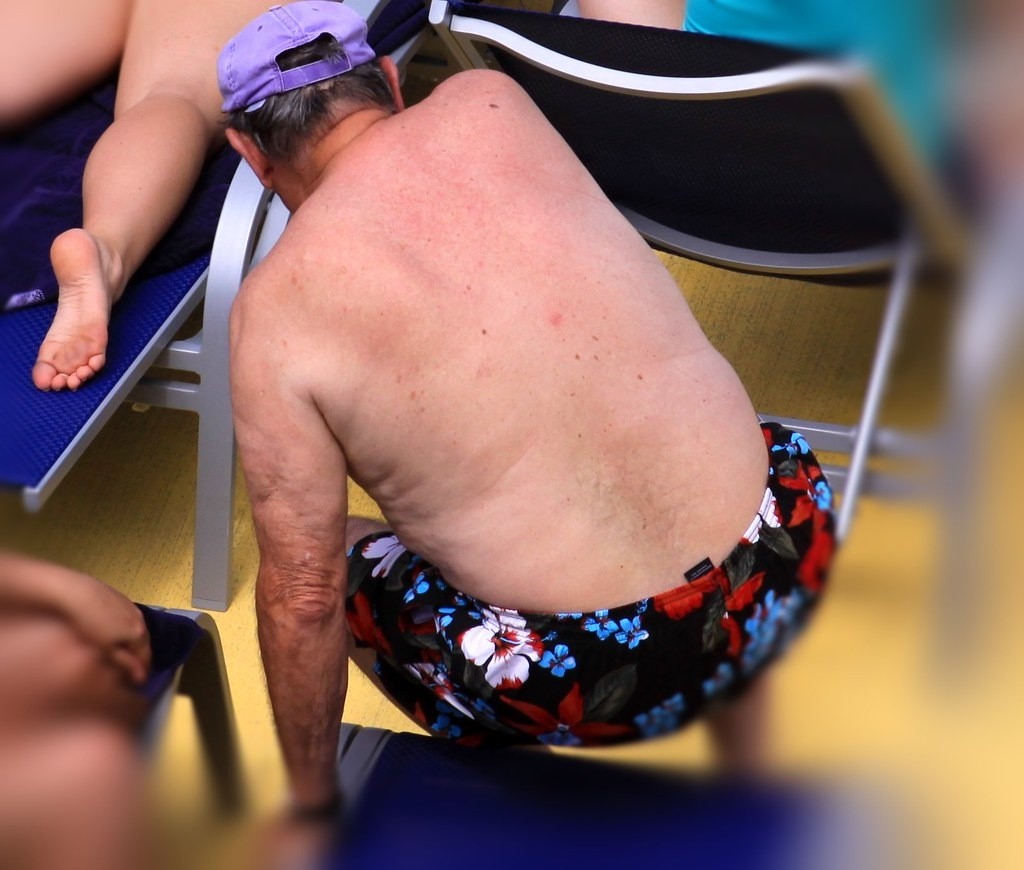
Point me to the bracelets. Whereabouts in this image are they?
[290,797,343,822]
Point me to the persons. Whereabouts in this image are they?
[0,553,152,869]
[0,0,295,394]
[579,1,944,188]
[215,1,839,870]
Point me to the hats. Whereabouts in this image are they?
[216,0,377,113]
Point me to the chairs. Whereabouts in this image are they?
[2,2,423,601]
[428,1,1023,672]
[131,601,248,832]
[313,721,860,870]
